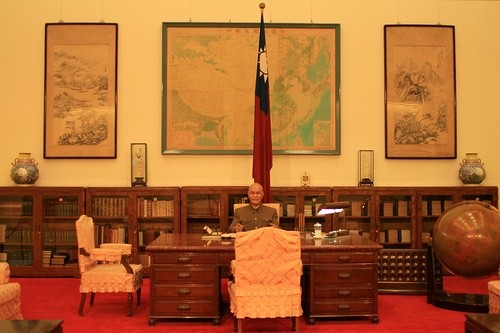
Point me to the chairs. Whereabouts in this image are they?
[226,227,304,333]
[75,215,144,316]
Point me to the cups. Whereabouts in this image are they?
[313,222,322,237]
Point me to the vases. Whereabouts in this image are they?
[458,153,486,184]
[9,153,39,186]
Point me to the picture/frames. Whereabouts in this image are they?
[383,24,457,160]
[161,21,341,155]
[44,21,117,159]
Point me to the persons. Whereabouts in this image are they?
[229,184,279,233]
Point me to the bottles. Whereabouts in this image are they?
[458,153,485,185]
[10,152,39,186]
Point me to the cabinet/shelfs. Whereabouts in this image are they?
[146,232,383,327]
[0,186,499,277]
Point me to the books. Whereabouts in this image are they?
[91,198,126,217]
[0,200,33,216]
[42,200,79,216]
[2,249,34,266]
[94,223,172,267]
[234,202,322,217]
[42,248,78,267]
[137,197,173,216]
[348,200,453,243]
[5,229,76,243]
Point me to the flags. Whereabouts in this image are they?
[252,16,273,203]
